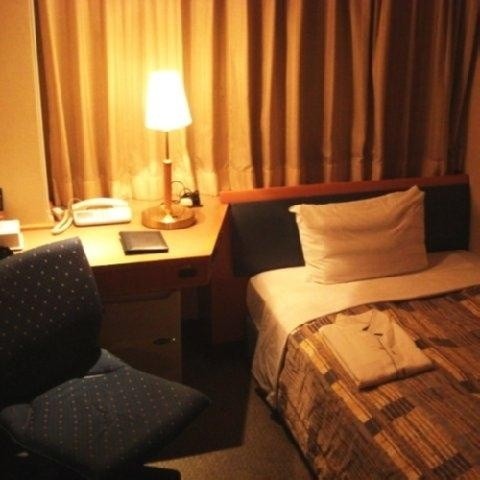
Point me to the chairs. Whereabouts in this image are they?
[0,236,207,480]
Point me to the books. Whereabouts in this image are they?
[118,229,168,254]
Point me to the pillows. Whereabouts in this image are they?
[289,185,432,284]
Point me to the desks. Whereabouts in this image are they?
[12,202,232,383]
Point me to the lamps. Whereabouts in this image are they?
[142,70,196,230]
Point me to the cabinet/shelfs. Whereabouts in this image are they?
[99,291,183,382]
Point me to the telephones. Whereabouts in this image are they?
[50,197,132,235]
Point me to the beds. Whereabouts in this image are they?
[246,248,479,480]
